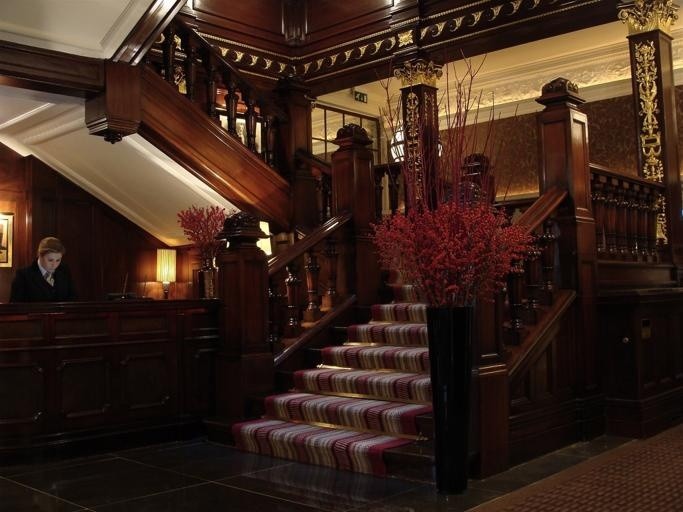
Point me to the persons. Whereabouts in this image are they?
[9,236,77,302]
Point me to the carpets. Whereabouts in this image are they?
[467,415,683,511]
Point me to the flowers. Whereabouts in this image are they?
[358,48,551,306]
[176,201,236,261]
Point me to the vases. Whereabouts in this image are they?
[426,304,478,501]
[198,261,219,301]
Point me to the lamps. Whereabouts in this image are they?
[156,248,177,292]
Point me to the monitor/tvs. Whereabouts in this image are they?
[106,293,137,300]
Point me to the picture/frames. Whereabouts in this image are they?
[0,211,15,269]
